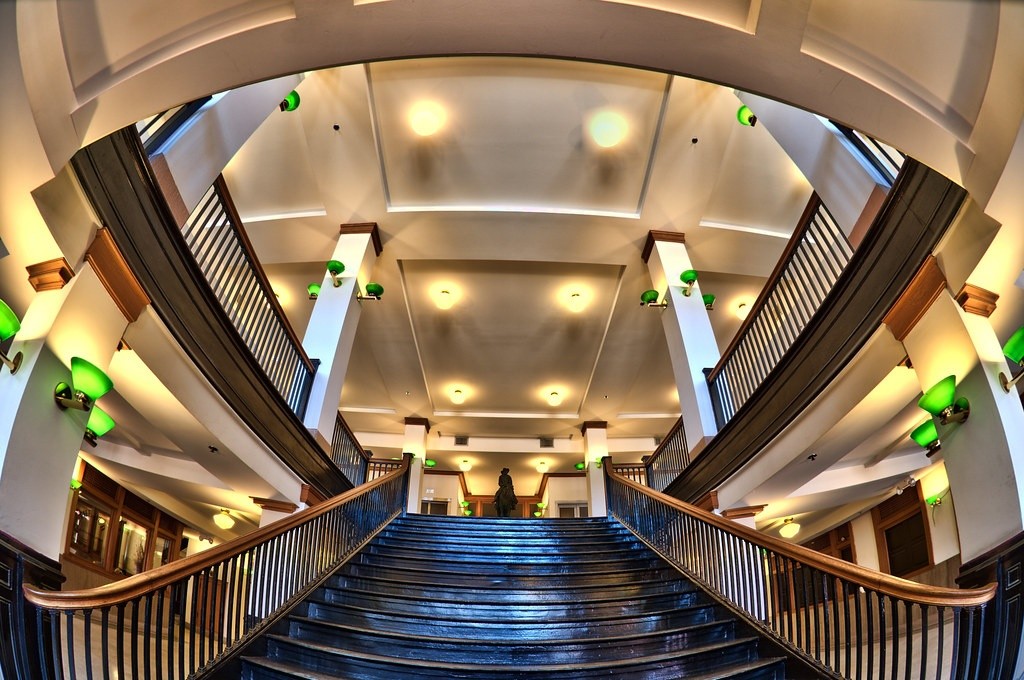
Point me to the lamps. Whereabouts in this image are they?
[356,283,384,301]
[736,105,757,127]
[576,458,603,472]
[459,501,472,516]
[702,295,715,310]
[327,261,345,287]
[213,509,235,530]
[926,495,942,506]
[641,290,667,309]
[680,270,698,297]
[779,517,800,538]
[83,405,116,448]
[307,282,321,299]
[911,419,941,458]
[70,478,83,491]
[280,90,301,112]
[998,326,1024,393]
[533,503,549,517]
[0,298,24,376]
[54,356,114,412]
[918,374,970,425]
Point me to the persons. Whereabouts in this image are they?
[493,468,518,511]
